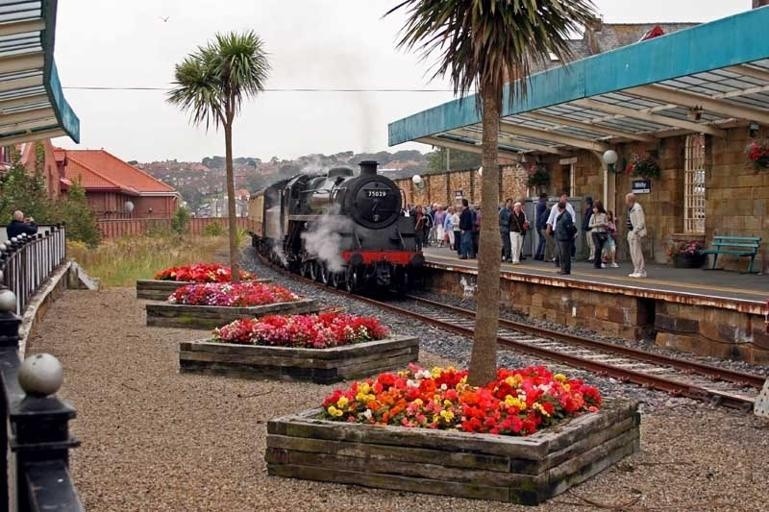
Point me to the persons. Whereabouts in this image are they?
[625,192,649,278]
[404,192,619,275]
[6,210,39,241]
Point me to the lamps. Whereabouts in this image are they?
[412,174,425,189]
[602,150,626,174]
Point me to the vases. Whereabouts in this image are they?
[672,253,690,268]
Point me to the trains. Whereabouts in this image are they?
[247,158,425,301]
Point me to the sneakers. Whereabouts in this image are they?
[557,270,570,274]
[588,255,619,269]
[628,272,647,278]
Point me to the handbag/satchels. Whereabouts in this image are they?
[596,232,608,242]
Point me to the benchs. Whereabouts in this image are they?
[700,236,761,275]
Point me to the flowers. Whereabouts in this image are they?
[742,136,769,170]
[153,262,300,307]
[663,239,706,258]
[211,311,392,349]
[623,150,660,181]
[523,155,551,188]
[322,361,603,435]
